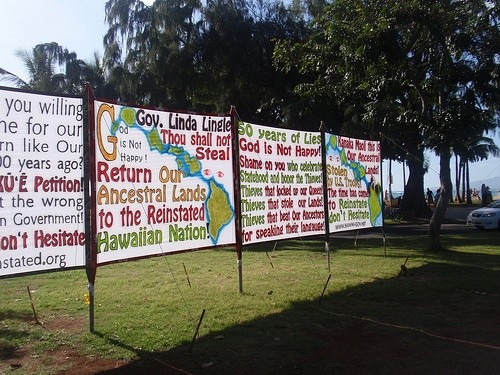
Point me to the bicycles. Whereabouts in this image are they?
[383,206,403,222]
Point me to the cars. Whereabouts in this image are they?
[466,199,500,230]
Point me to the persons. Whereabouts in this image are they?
[472,186,492,201]
[425,187,442,208]
[384,189,389,201]
